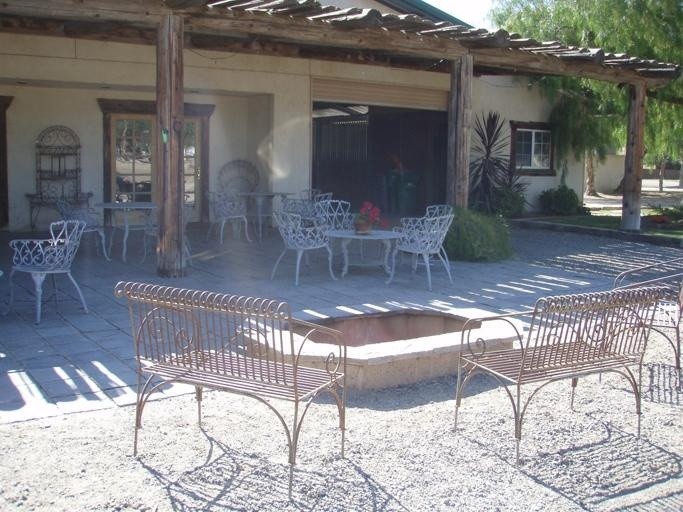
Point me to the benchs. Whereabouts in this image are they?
[454,286,662,440]
[114,281,346,464]
[612,257,683,369]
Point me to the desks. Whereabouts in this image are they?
[94,202,157,265]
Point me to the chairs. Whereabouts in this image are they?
[139,201,196,266]
[205,188,455,293]
[0,220,88,325]
[56,200,113,263]
